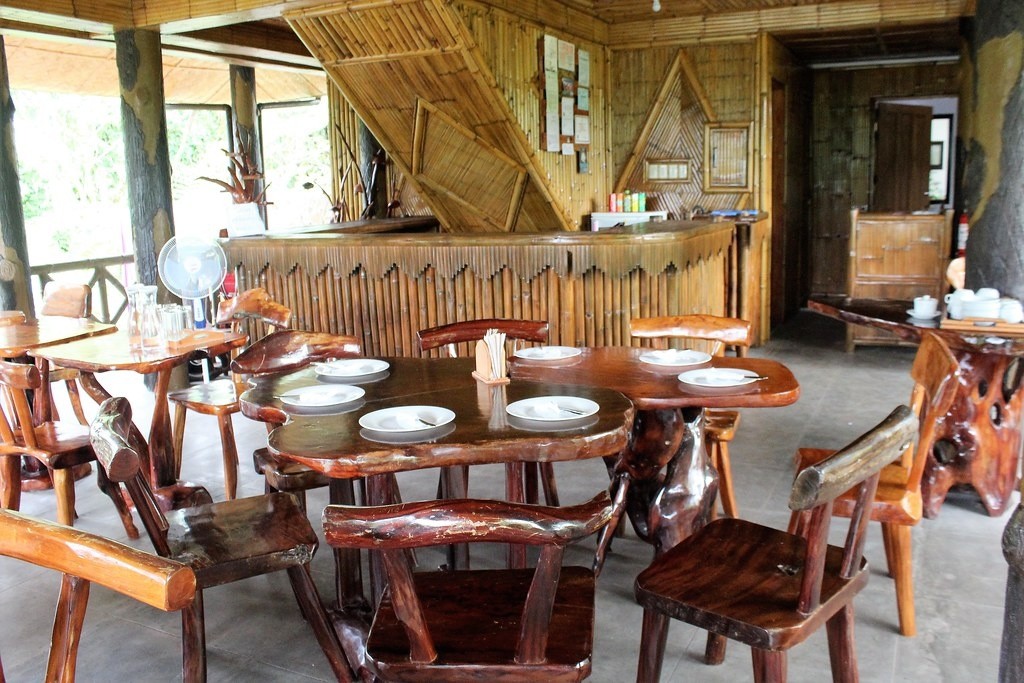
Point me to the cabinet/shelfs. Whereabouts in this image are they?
[590,210,668,231]
[845,206,954,354]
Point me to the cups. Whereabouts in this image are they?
[123,282,193,352]
[913,296,938,316]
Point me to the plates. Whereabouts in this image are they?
[678,382,760,396]
[357,405,456,433]
[279,384,366,407]
[314,358,390,377]
[315,369,390,385]
[506,396,601,421]
[507,414,600,432]
[280,398,366,417]
[639,348,713,366]
[513,355,582,370]
[515,345,582,361]
[359,422,457,445]
[906,308,942,320]
[639,362,713,375]
[677,367,760,388]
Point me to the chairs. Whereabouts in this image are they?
[40,285,93,426]
[0,360,140,541]
[90,395,356,683]
[0,508,196,683]
[610,312,751,541]
[322,470,630,683]
[786,331,961,638]
[418,319,563,507]
[168,286,292,501]
[633,403,921,683]
[229,327,420,571]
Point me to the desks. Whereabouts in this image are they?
[0,315,122,425]
[25,326,252,488]
[239,344,801,683]
[807,293,1024,522]
[0,310,26,326]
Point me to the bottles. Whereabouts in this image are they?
[944,287,1023,326]
[609,189,646,212]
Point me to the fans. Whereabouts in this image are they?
[157,233,229,384]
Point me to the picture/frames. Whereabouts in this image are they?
[704,120,754,194]
[930,141,943,170]
[642,157,693,184]
